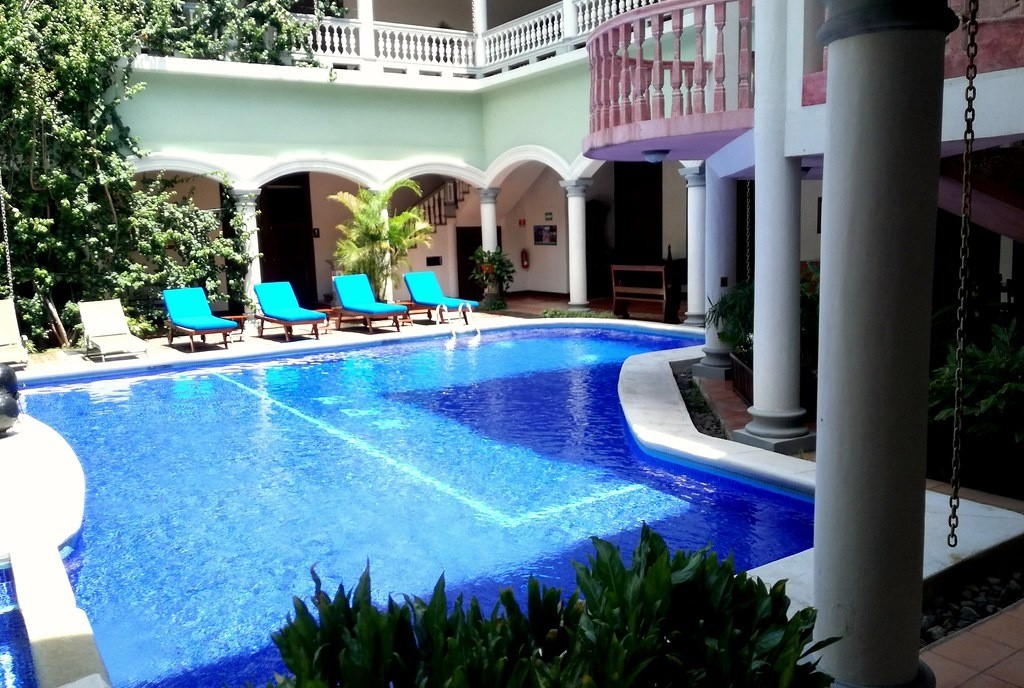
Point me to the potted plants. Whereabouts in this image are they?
[701,260,820,419]
[468,245,516,294]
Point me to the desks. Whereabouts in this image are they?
[310,309,335,335]
[395,300,425,324]
[222,316,250,341]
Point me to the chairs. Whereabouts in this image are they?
[0,299,29,370]
[253,281,326,341]
[163,287,238,352]
[78,299,149,362]
[332,275,408,333]
[402,271,479,324]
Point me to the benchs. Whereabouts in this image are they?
[612,265,686,323]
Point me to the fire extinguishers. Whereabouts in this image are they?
[521,249,529,269]
